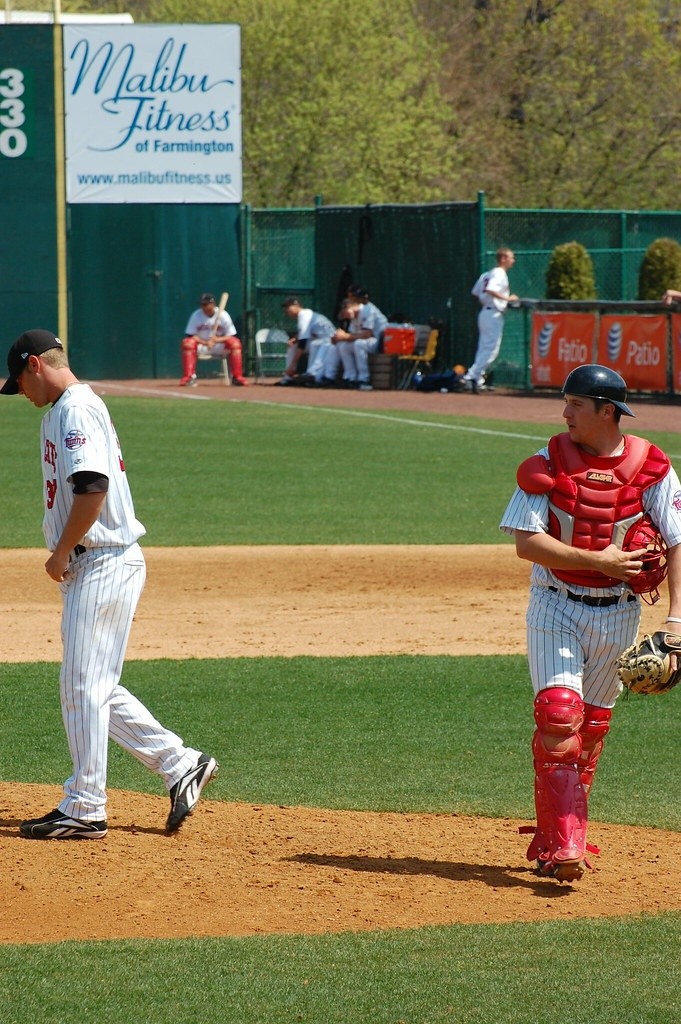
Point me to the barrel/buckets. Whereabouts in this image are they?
[383,322,415,355]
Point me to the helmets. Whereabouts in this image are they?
[561,364,636,418]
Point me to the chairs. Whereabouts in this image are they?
[254,328,292,386]
[196,341,232,386]
[398,329,439,391]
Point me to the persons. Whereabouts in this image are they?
[0,331,221,838]
[499,365,681,883]
[274,299,335,385]
[465,250,519,396]
[180,293,248,386]
[320,284,387,390]
[661,289,681,305]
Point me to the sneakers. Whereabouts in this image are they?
[165,752,220,832]
[20,809,107,840]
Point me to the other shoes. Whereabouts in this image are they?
[274,372,371,391]
[232,376,246,386]
[184,378,198,388]
[539,859,586,883]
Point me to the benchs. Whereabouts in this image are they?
[298,323,430,391]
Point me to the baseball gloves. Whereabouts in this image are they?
[613,622,681,702]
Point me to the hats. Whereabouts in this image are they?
[201,297,216,304]
[0,330,63,396]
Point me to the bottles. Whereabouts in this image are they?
[191,370,198,388]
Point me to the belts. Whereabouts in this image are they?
[486,306,494,309]
[68,544,87,563]
[550,584,636,607]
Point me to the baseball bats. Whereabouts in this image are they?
[208,292,229,350]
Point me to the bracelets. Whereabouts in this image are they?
[666,617,681,622]
